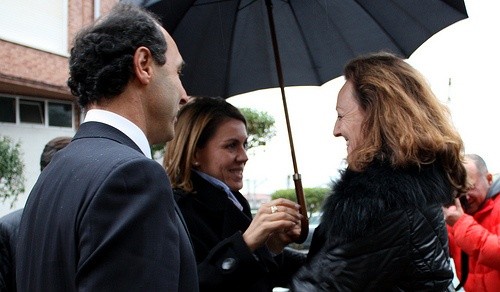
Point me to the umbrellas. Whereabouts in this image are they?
[118,0,469,245]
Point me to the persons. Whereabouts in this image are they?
[162,96,304,292]
[284,51,468,292]
[0,135,72,292]
[15,1,199,292]
[441,153,500,292]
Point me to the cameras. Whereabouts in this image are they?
[457,187,467,205]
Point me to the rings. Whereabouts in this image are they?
[271,206,280,214]
